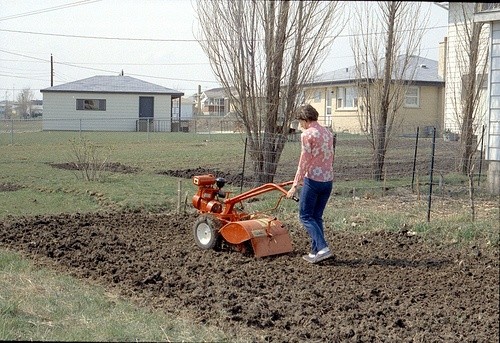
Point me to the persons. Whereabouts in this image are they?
[285,105,335,263]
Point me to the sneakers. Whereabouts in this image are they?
[309,249,334,263]
[302,255,315,262]
